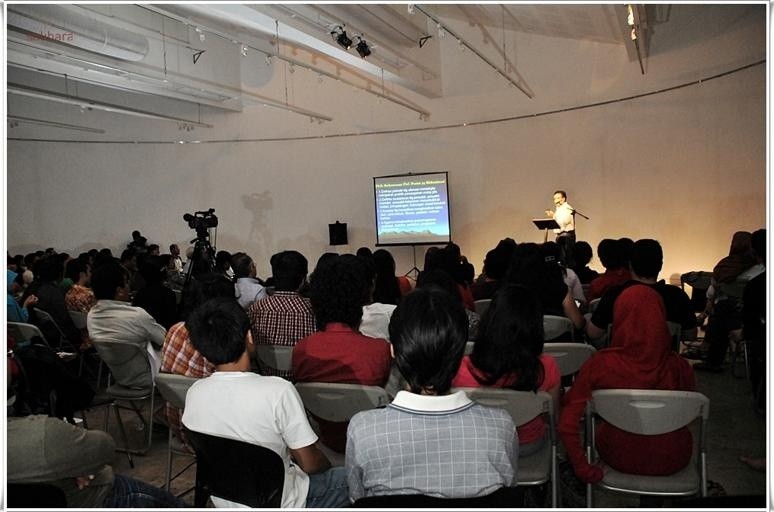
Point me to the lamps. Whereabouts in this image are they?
[337,33,352,50]
[356,40,373,59]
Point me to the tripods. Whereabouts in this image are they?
[174,238,230,318]
[401,266,422,284]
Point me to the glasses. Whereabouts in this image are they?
[6,348,15,359]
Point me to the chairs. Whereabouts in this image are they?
[681,271,713,314]
[254,342,294,374]
[9,322,86,403]
[450,387,558,510]
[545,342,597,380]
[69,308,92,334]
[154,372,203,495]
[33,308,70,339]
[92,338,156,457]
[294,381,389,466]
[184,422,286,510]
[20,343,137,467]
[584,388,712,506]
[544,315,576,343]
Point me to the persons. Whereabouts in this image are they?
[160,293,216,379]
[587,239,633,302]
[7,415,195,508]
[293,263,391,455]
[557,285,694,482]
[450,283,561,456]
[359,264,398,358]
[737,454,766,474]
[567,241,599,282]
[518,243,586,329]
[133,262,177,330]
[22,256,72,333]
[587,239,698,342]
[86,264,167,386]
[546,191,575,243]
[345,285,519,504]
[181,297,350,507]
[7,230,170,275]
[65,261,97,351]
[736,229,766,419]
[211,251,233,283]
[702,231,765,373]
[169,244,182,275]
[374,249,412,305]
[7,269,38,323]
[246,250,319,383]
[545,242,586,301]
[413,233,518,311]
[230,253,268,311]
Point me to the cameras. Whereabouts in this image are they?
[182,207,218,244]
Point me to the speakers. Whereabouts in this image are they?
[328,221,349,247]
